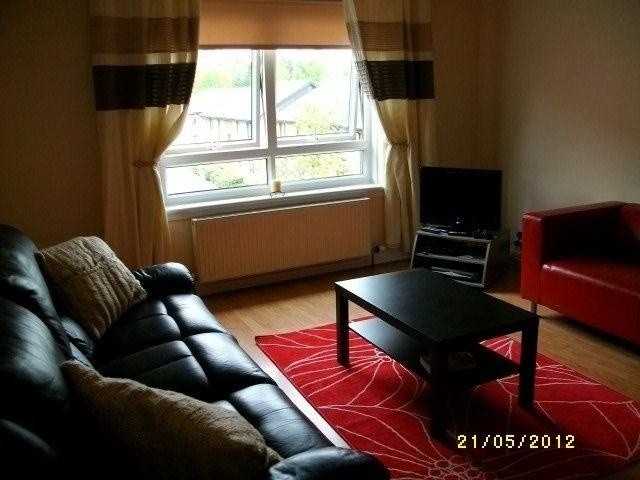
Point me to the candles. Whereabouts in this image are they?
[270,179,282,192]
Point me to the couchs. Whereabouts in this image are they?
[0,225,392,480]
[519,199,639,347]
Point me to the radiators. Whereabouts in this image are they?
[190,197,386,284]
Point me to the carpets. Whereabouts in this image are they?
[253,316,639,480]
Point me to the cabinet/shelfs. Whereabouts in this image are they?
[408,226,510,290]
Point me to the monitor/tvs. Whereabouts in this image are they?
[420,166,502,236]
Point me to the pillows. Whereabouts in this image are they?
[33,234,149,341]
[59,359,282,480]
